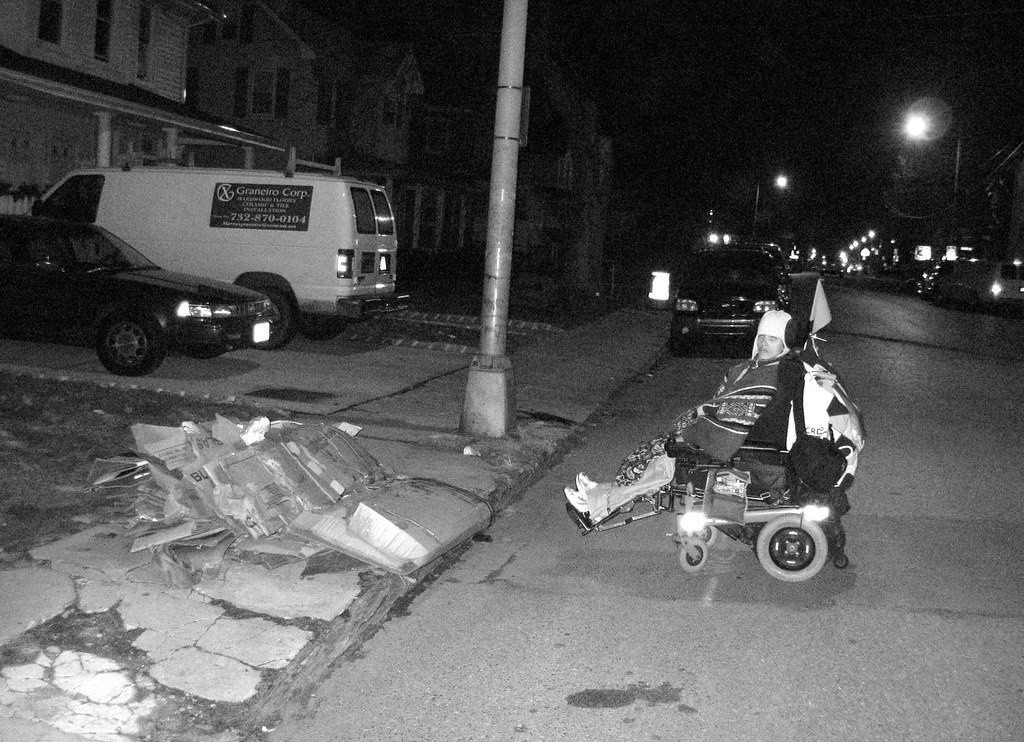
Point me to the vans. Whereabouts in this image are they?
[20,146,413,342]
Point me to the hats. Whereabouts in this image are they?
[751,309,792,360]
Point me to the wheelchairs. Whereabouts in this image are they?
[565,311,850,582]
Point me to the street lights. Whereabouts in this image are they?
[901,111,962,227]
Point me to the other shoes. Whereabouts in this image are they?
[564,487,591,517]
[575,471,598,492]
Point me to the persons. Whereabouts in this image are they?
[562,310,793,530]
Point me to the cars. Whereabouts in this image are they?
[860,257,1024,315]
[0,213,279,377]
[670,247,790,352]
